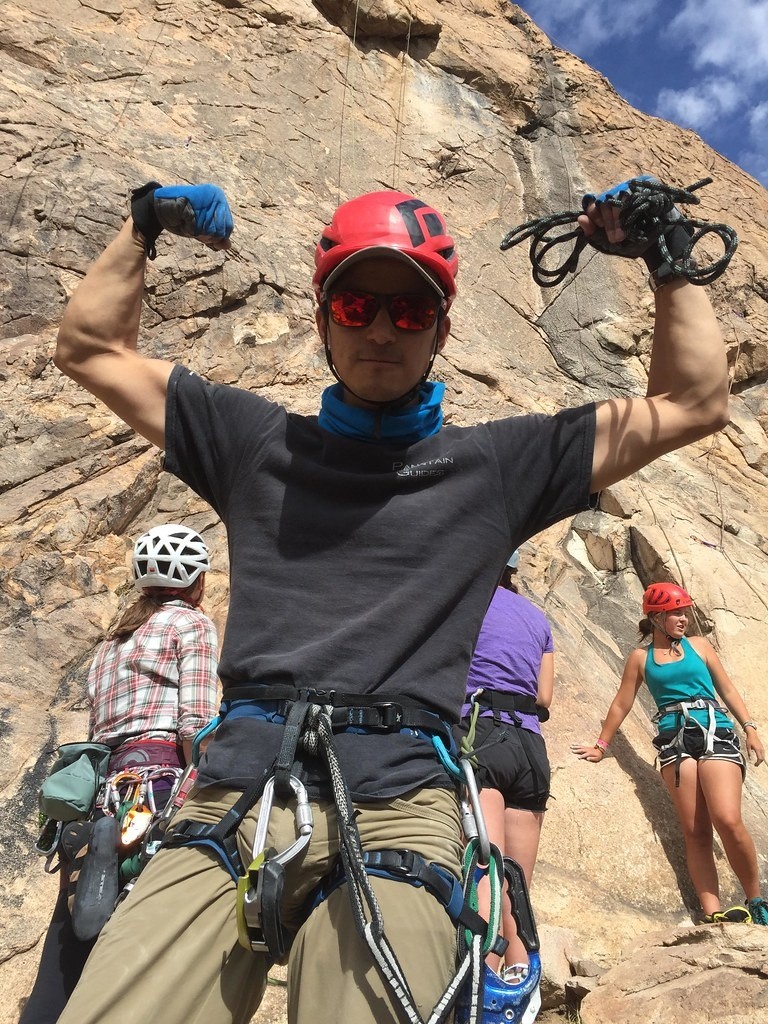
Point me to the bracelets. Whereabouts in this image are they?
[646,257,699,292]
[594,739,609,754]
[741,721,757,732]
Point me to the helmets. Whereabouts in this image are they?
[506,548,520,569]
[130,523,213,587]
[310,189,459,304]
[642,584,694,615]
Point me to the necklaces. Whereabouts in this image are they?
[661,646,671,655]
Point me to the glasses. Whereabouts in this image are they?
[326,287,440,331]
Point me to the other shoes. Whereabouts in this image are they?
[748,896,768,926]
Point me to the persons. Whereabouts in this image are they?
[53,176,729,1024]
[459,547,556,989]
[18,523,221,1024]
[570,583,768,927]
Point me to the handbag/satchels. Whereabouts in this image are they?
[37,742,112,823]
